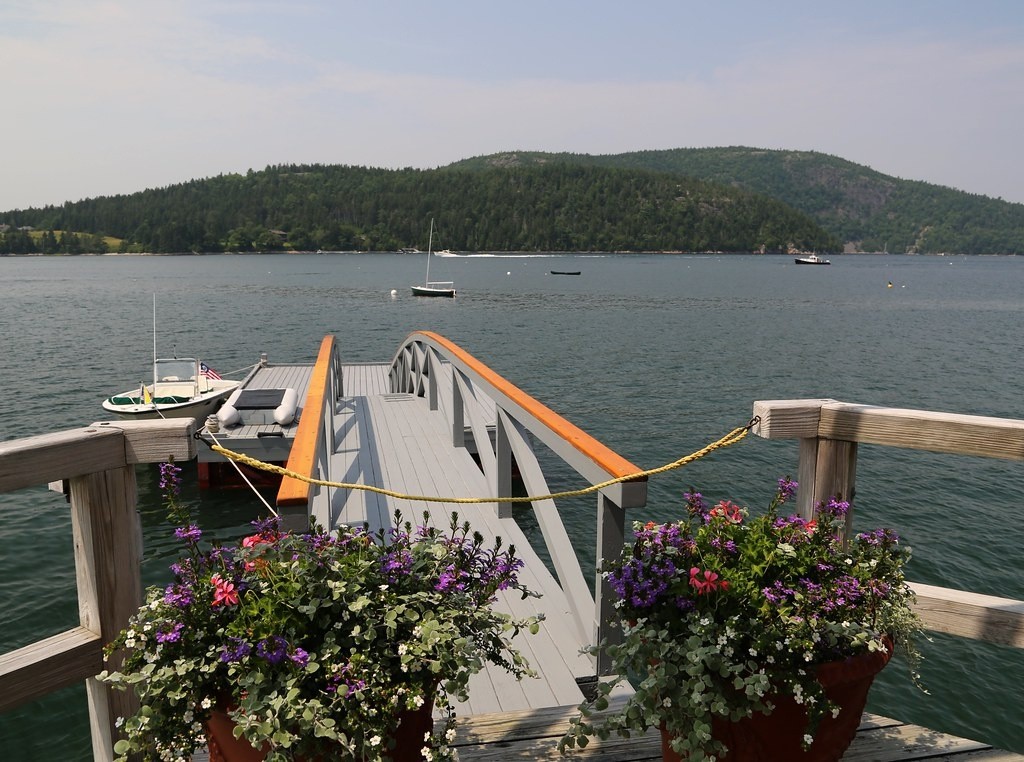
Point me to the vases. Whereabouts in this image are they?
[628,618,896,762]
[194,684,436,762]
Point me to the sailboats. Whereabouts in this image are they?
[410,216,457,299]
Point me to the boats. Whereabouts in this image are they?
[100,291,242,430]
[550,270,581,275]
[216,386,298,428]
[794,246,832,265]
[433,249,456,257]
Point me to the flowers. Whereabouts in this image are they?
[95,456,549,762]
[558,474,933,762]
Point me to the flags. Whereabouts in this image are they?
[199,361,223,380]
[140,382,151,405]
